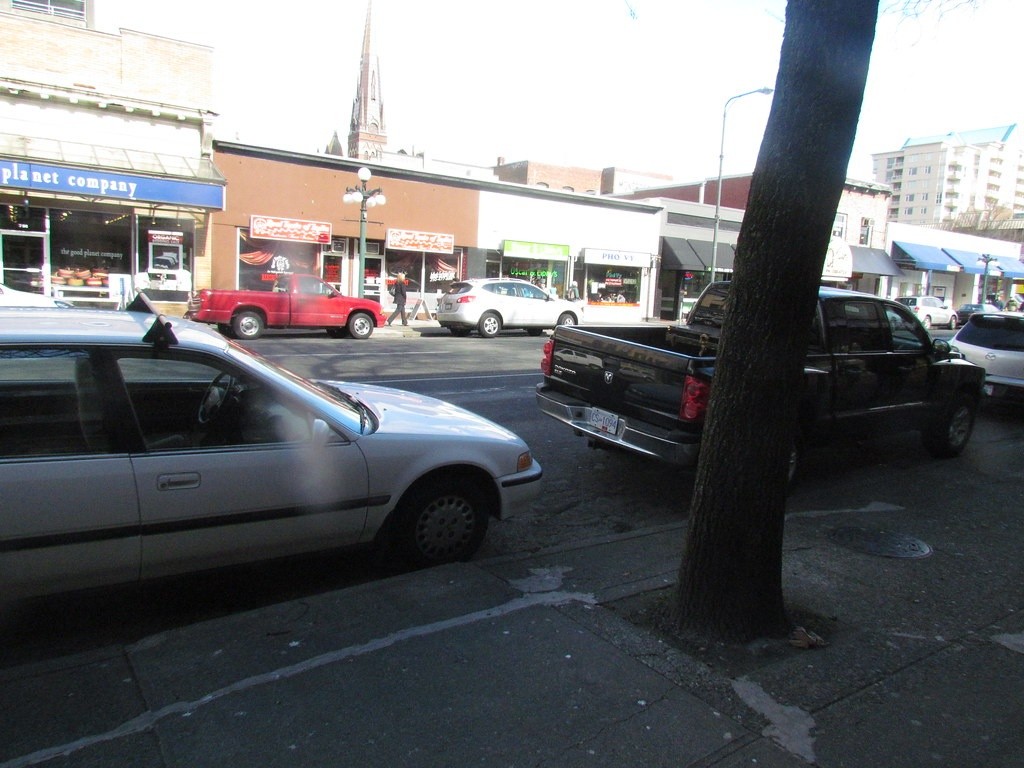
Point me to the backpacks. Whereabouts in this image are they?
[567,287,575,301]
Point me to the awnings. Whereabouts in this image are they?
[849,246,906,277]
[941,248,1001,276]
[687,239,735,273]
[893,241,960,272]
[991,255,1024,278]
[661,236,704,271]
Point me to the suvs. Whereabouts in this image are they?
[437,278,582,338]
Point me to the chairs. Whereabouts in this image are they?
[74,358,188,453]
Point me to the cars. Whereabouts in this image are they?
[956,304,1000,327]
[0,281,75,308]
[944,310,1024,412]
[895,296,958,332]
[0,286,546,601]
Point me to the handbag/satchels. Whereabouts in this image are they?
[389,283,396,297]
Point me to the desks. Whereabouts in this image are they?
[52,284,119,308]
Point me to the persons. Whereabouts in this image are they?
[1006,297,1020,312]
[571,281,581,301]
[387,274,409,327]
[1018,299,1024,312]
[994,295,1004,311]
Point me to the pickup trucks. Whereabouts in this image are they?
[536,282,989,493]
[182,273,388,340]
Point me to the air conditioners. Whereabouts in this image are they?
[865,219,875,227]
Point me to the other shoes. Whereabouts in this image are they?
[402,323,408,326]
[389,322,391,326]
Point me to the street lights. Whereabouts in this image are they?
[976,253,1001,304]
[342,166,387,299]
[709,85,776,282]
